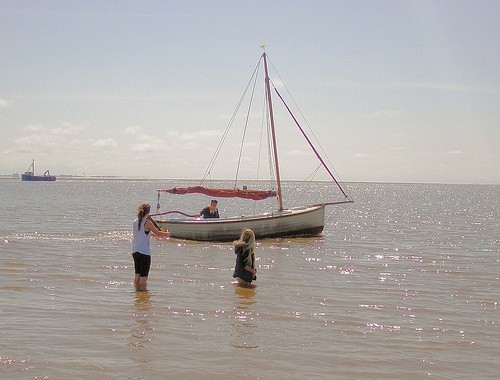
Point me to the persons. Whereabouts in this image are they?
[131,203,171,292]
[233,227,258,287]
[195,199,219,219]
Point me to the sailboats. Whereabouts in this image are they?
[151,44,353,242]
[21,159,56,182]
